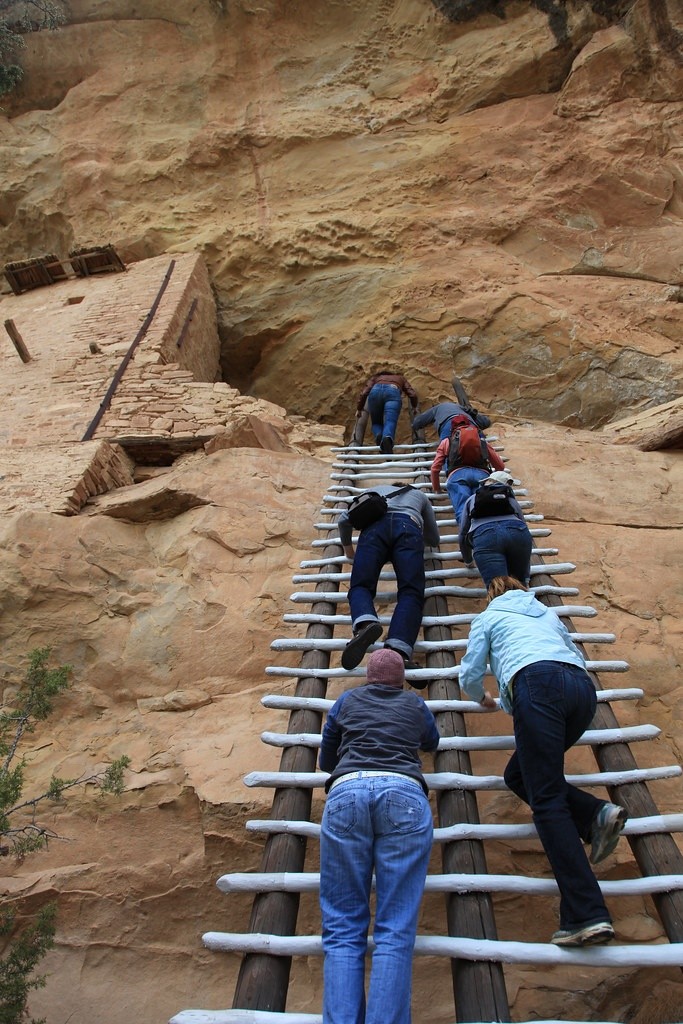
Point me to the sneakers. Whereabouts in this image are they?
[550,919,614,947]
[588,802,628,864]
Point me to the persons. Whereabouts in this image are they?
[320,648,440,1024]
[458,575,628,947]
[458,471,533,592]
[338,482,440,690]
[413,402,491,478]
[431,415,505,524]
[355,371,418,462]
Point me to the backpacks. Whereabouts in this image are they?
[469,482,519,520]
[444,425,492,473]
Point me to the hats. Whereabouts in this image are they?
[451,414,470,430]
[367,650,405,688]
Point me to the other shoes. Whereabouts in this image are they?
[348,490,387,530]
[376,433,383,445]
[380,437,394,462]
[403,659,428,690]
[341,622,384,670]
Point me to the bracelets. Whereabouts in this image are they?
[482,694,485,700]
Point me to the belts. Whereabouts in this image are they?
[328,770,423,790]
